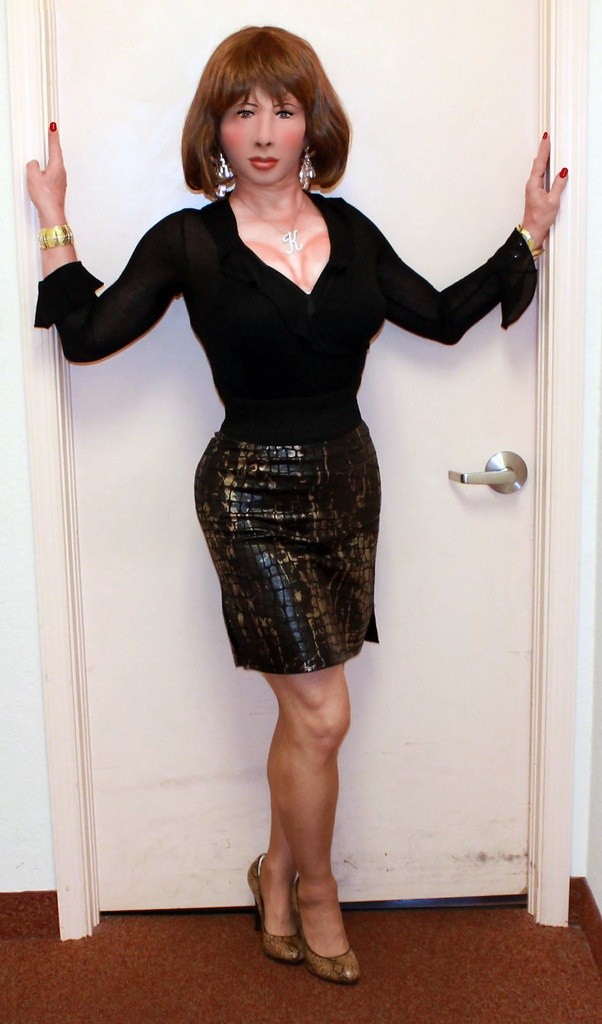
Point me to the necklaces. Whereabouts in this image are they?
[236,190,306,254]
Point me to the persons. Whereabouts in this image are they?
[23,23,573,985]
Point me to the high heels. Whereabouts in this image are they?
[247,852,305,966]
[291,876,362,985]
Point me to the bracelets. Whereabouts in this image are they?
[516,223,545,260]
[39,224,74,251]
